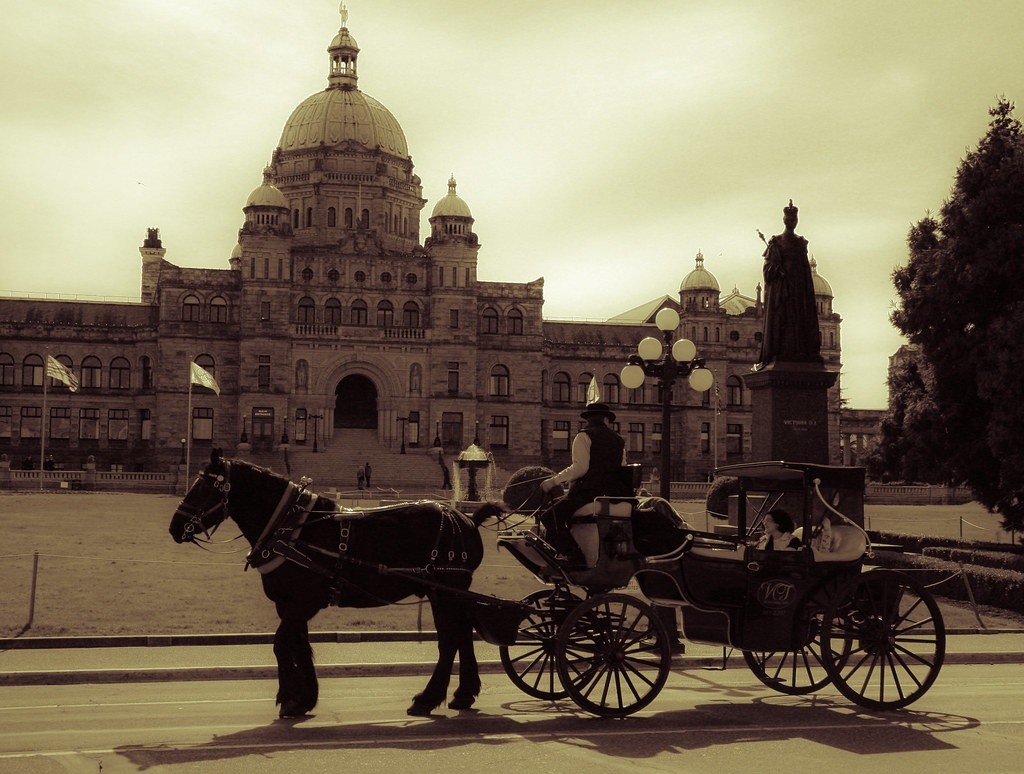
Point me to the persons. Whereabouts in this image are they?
[539,403,628,569]
[759,198,826,363]
[356,464,365,490]
[441,467,452,490]
[364,463,372,487]
[754,508,803,550]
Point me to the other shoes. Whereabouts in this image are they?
[554,550,588,568]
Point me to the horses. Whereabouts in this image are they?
[170,447,509,720]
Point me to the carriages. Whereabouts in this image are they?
[168,459,987,718]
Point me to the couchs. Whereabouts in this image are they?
[634,496,866,609]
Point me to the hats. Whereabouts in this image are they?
[580,404,616,421]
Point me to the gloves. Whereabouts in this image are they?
[539,476,561,493]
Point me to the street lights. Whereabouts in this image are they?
[620,307,715,504]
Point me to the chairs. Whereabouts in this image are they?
[568,464,642,565]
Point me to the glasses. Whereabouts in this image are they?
[761,520,776,523]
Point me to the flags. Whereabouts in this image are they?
[47,354,80,393]
[190,362,221,397]
[585,375,602,408]
